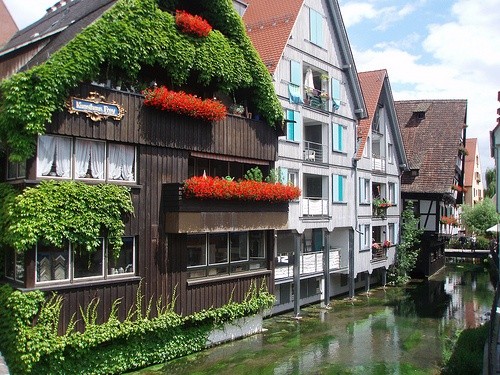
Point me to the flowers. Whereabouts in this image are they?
[372,243,379,249]
[372,198,392,208]
[453,185,463,192]
[181,174,302,203]
[175,9,211,38]
[143,85,227,122]
[440,216,457,224]
[383,241,391,247]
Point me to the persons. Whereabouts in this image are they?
[457,233,467,251]
[471,234,477,252]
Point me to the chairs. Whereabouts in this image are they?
[304,150,315,161]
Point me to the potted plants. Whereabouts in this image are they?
[230,105,245,115]
